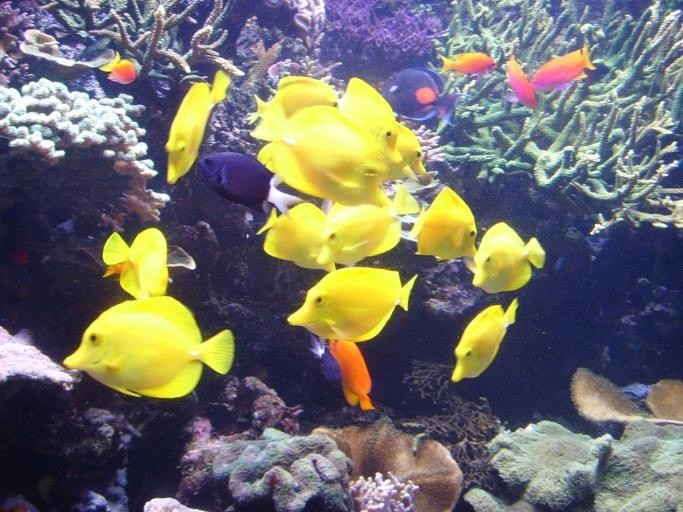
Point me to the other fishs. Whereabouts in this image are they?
[62,44,596,412]
